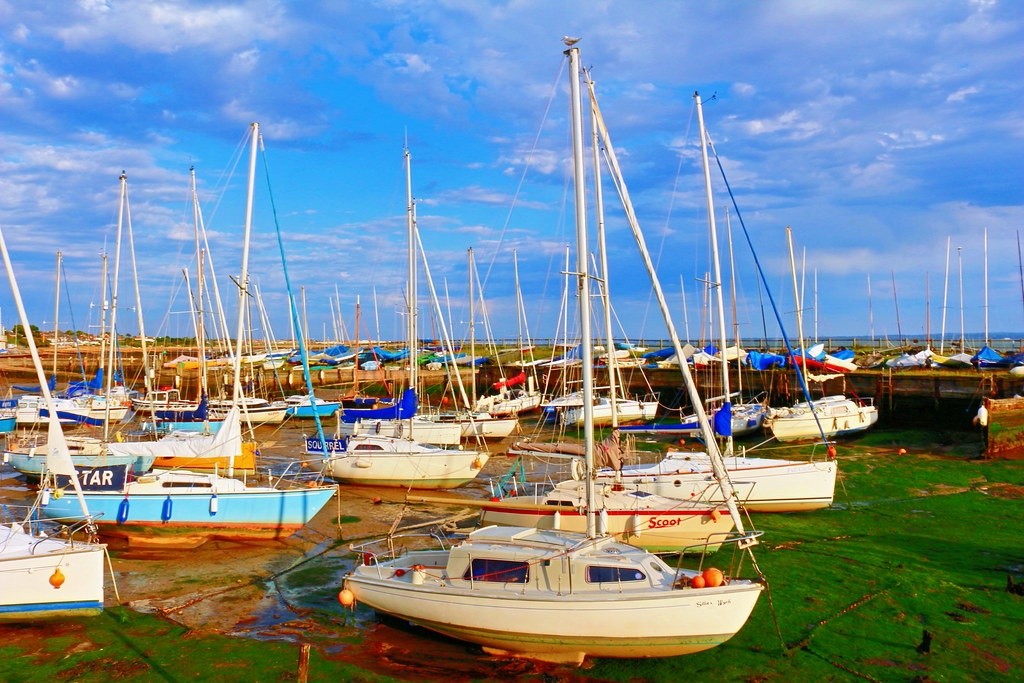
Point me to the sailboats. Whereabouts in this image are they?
[1,227,121,627]
[32,168,340,536]
[336,43,773,669]
[2,82,1024,564]
